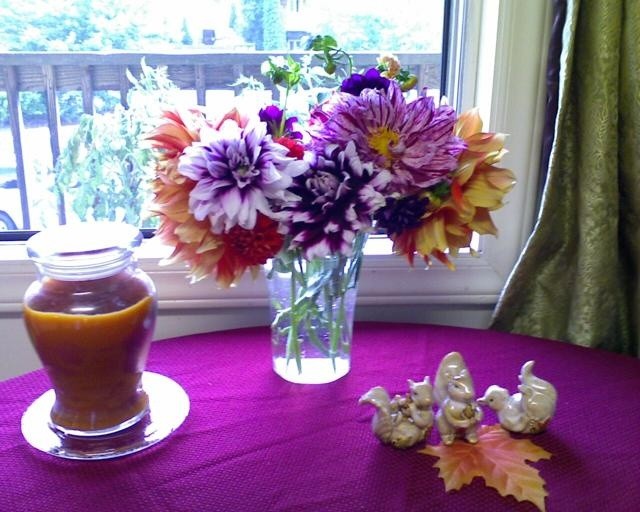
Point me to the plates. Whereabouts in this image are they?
[21,371,191,462]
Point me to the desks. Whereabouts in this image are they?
[1,320,639,512]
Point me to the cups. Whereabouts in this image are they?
[24,222,156,437]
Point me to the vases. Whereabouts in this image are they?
[264,234,367,383]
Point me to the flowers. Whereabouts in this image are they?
[145,34,517,373]
